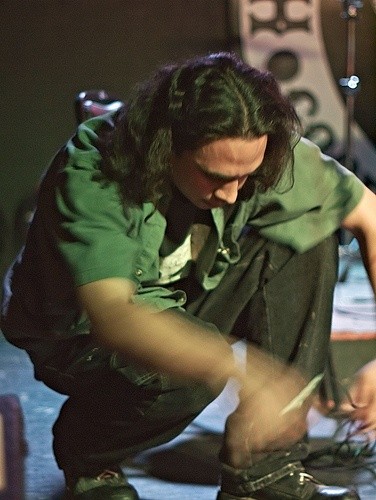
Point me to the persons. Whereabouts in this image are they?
[0,51,376,500]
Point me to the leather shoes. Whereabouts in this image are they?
[216,471,361,500]
[53,440,140,500]
